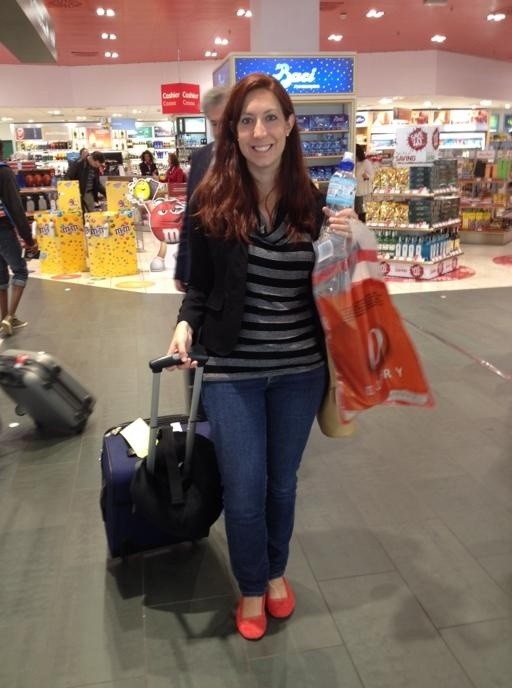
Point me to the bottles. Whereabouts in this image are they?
[375,228,460,263]
[319,158,357,242]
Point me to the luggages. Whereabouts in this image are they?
[0,349,96,438]
[101,352,224,562]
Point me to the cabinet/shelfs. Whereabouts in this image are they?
[17,116,208,232]
[287,94,512,279]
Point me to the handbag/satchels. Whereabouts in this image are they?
[316,336,356,437]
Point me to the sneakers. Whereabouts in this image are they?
[0,315,28,335]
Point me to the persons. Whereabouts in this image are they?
[166,73,358,639]
[174,86,235,412]
[356,145,374,214]
[0,151,193,334]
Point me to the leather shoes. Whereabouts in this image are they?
[235,593,267,640]
[266,576,295,618]
